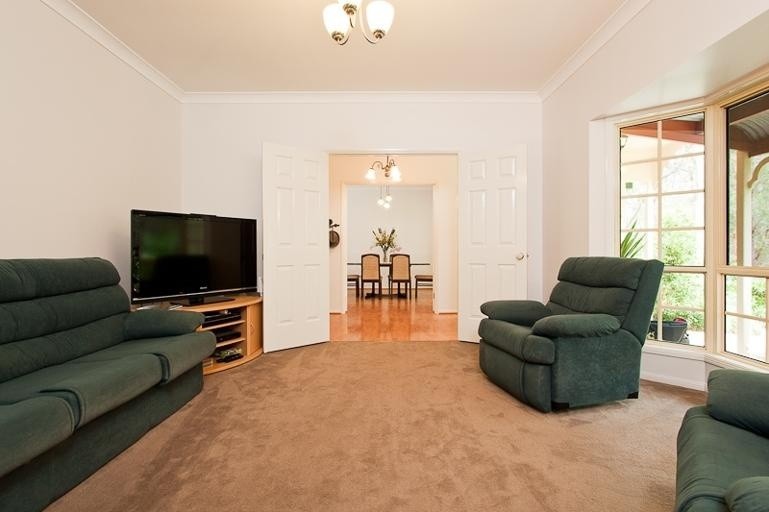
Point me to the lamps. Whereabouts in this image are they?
[323,0,394,45]
[366,155,401,209]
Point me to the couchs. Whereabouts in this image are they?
[0,257,216,512]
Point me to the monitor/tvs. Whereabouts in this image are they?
[131,209,257,307]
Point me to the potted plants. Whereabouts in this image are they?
[369,226,398,262]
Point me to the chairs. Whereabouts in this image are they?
[347,275,359,297]
[361,253,383,300]
[388,254,411,300]
[479,257,663,414]
[674,370,769,512]
[415,275,433,298]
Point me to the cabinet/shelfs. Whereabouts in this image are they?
[132,295,262,375]
